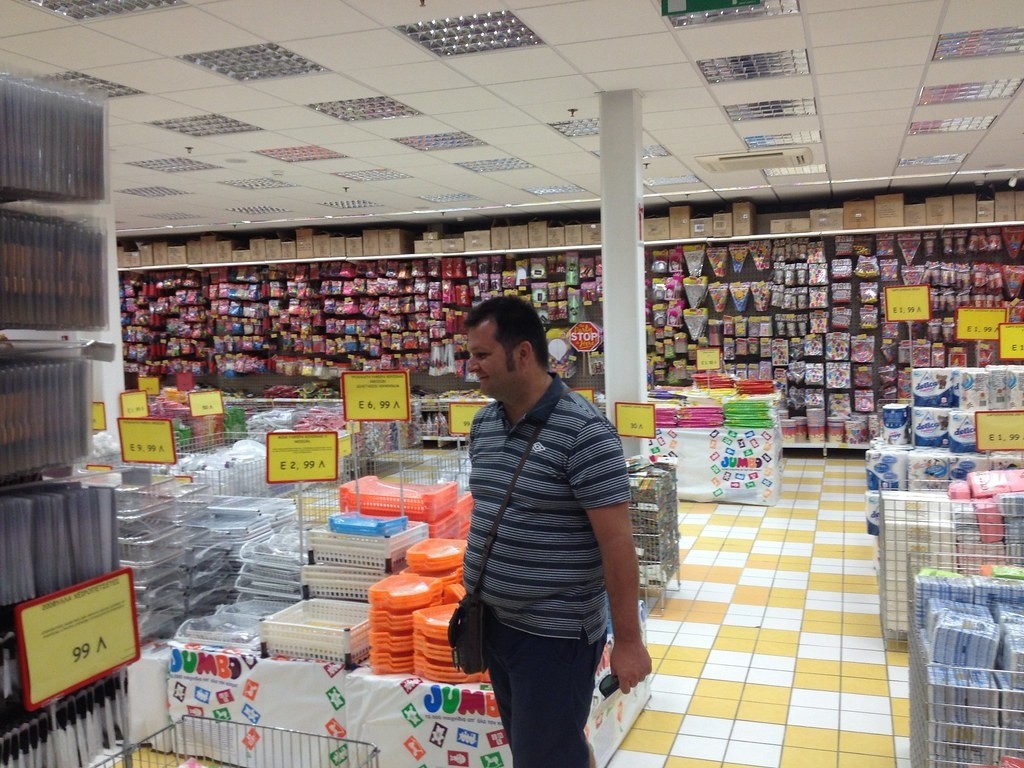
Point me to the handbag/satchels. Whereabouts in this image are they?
[449,592,486,677]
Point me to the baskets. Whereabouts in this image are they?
[257,474,473,663]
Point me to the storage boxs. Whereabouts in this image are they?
[117,190,1024,267]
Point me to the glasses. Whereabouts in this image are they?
[599,674,620,700]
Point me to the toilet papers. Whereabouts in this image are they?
[865,364,1024,538]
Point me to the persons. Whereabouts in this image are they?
[462,294,652,768]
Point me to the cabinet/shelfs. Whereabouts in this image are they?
[0,74,115,767]
[168,626,653,768]
[639,415,782,505]
